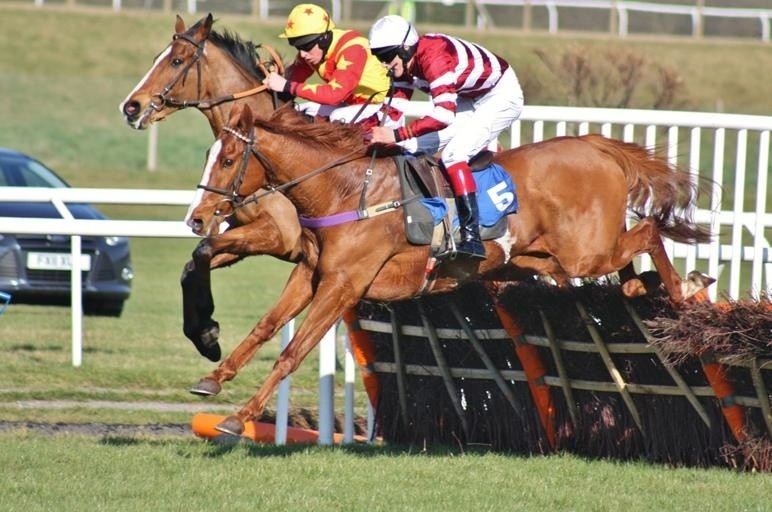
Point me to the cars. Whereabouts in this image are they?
[0,148,133,318]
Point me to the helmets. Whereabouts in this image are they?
[278,3,335,45]
[368,15,418,54]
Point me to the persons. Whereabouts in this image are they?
[362,15,529,263]
[261,6,389,126]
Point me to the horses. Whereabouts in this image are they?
[118,11,726,437]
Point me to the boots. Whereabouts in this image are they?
[436,161,487,262]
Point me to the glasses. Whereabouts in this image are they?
[295,39,320,51]
[376,46,398,64]
